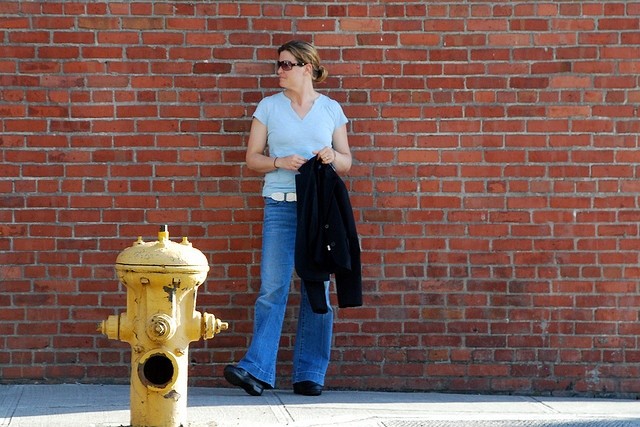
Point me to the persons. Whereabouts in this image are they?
[223,39,353,396]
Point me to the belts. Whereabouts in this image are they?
[268,193,297,203]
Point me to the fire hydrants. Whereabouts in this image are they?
[98,224,229,427]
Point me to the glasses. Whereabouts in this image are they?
[277,61,308,71]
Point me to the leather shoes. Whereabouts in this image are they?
[223,365,264,396]
[293,381,322,396]
